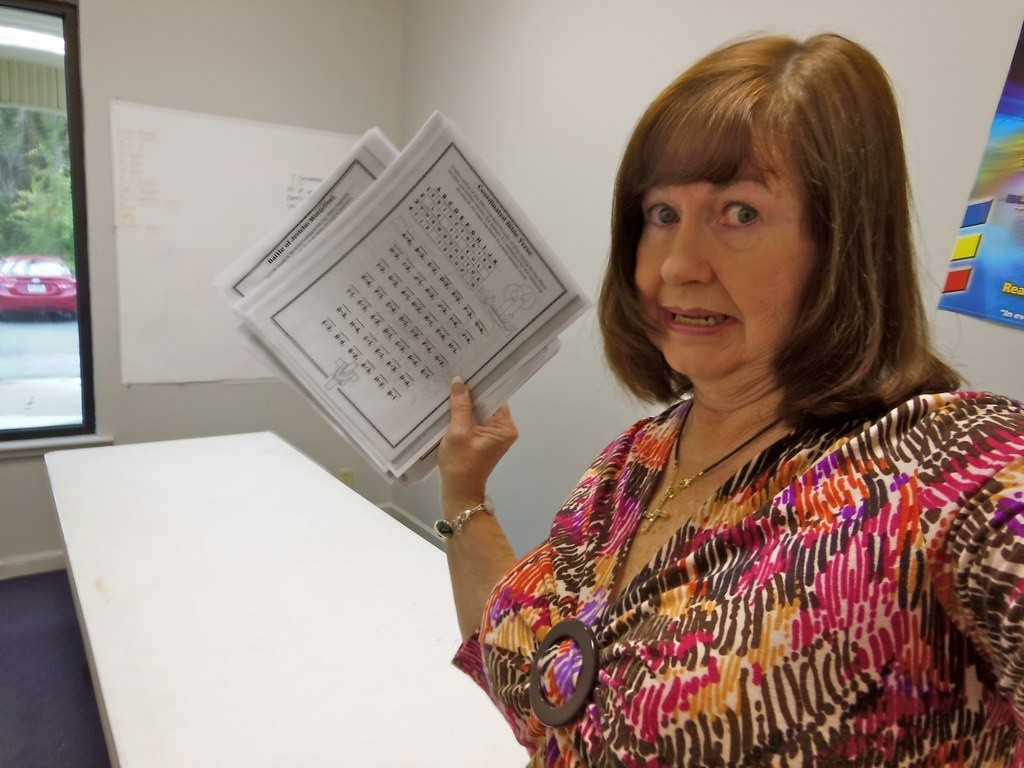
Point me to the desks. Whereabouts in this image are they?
[43,431,530,767]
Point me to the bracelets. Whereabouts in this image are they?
[435,493,495,539]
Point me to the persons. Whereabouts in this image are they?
[436,33,1024,768]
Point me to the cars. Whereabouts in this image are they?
[0,255,76,317]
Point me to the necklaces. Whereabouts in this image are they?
[638,400,787,535]
